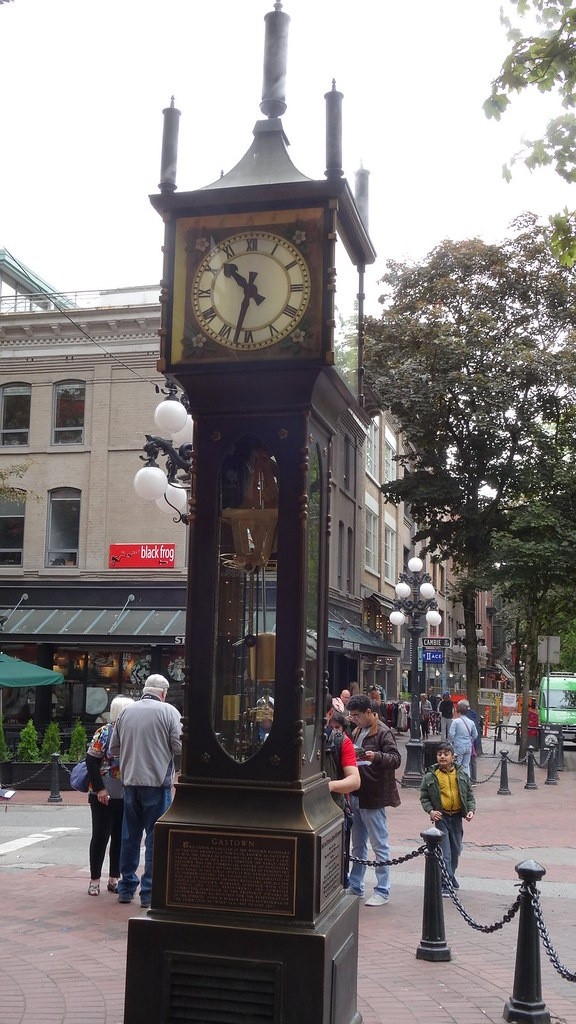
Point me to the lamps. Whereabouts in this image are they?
[339,619,350,634]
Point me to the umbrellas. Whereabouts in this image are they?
[0,651,64,687]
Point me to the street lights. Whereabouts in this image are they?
[389,557,442,788]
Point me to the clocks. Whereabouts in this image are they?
[191,231,313,351]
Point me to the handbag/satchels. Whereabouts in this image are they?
[70,757,91,793]
[472,743,477,760]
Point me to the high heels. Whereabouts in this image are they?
[106,879,120,894]
[88,879,102,897]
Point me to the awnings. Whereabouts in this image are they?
[1,608,277,646]
[328,620,402,657]
[495,664,515,681]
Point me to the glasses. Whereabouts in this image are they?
[348,711,363,720]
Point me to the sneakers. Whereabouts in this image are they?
[365,894,388,905]
[345,887,365,899]
[140,895,149,909]
[442,887,451,898]
[117,894,133,905]
[453,877,461,889]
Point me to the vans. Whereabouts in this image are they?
[538,672,576,744]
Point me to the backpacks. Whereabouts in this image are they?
[323,732,348,814]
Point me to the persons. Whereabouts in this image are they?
[420,742,476,898]
[437,691,454,742]
[419,693,433,740]
[109,674,185,908]
[86,694,136,895]
[346,695,401,907]
[448,704,478,778]
[458,699,482,788]
[333,687,381,738]
[324,692,361,894]
